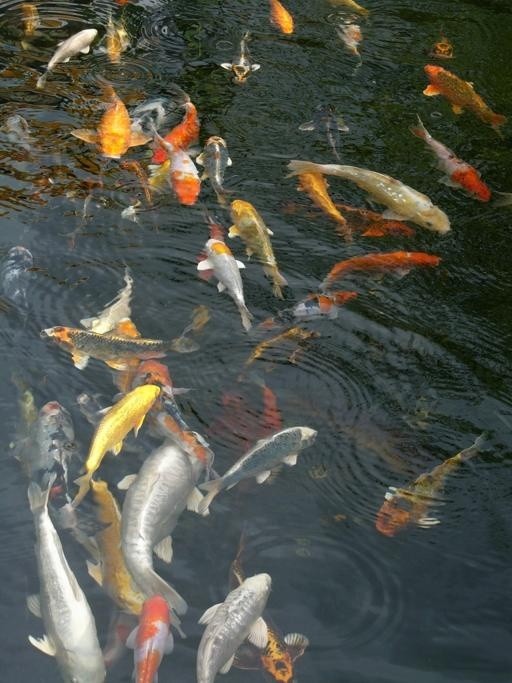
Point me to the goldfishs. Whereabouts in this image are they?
[0,2,369,682]
